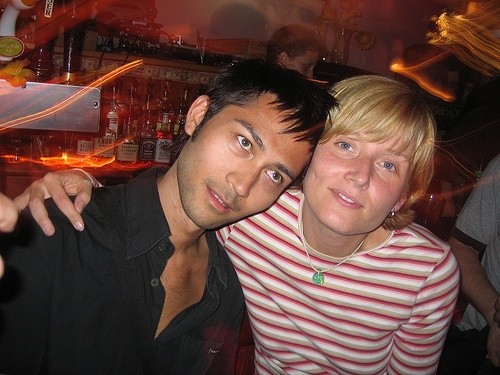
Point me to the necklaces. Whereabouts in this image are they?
[301,220,365,285]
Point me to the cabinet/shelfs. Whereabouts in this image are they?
[0,49,328,181]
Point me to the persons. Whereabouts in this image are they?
[0,25,500,375]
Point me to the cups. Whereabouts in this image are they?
[412,194,446,231]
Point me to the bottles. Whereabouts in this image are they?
[63,86,190,167]
